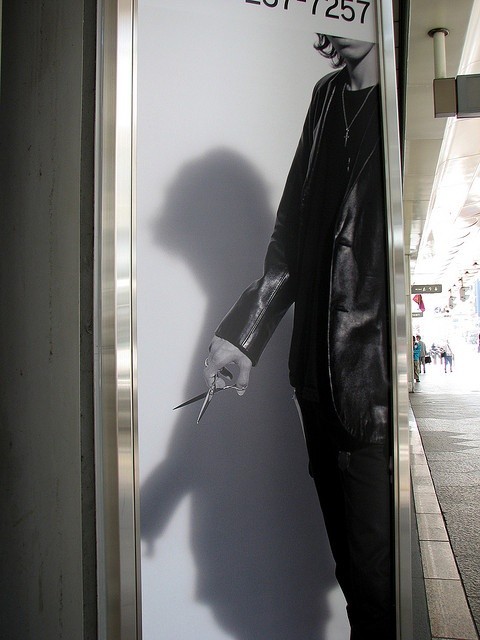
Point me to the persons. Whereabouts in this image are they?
[413,336,421,382]
[202,32,397,640]
[417,336,427,372]
[430,339,454,374]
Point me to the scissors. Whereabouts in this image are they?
[173,356,246,425]
